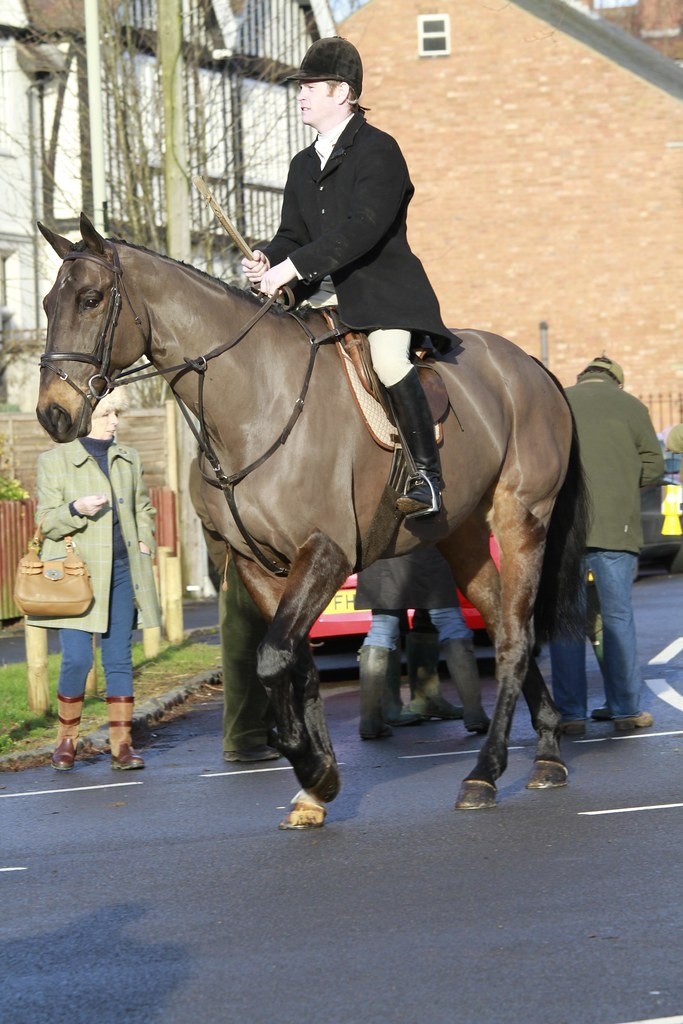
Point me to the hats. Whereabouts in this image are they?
[590,358,624,386]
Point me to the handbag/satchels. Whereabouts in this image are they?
[14,521,95,618]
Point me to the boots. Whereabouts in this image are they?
[105,694,144,769]
[406,632,464,720]
[442,638,491,733]
[384,651,422,726]
[357,646,392,739]
[52,691,84,771]
[384,367,443,516]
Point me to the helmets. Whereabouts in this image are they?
[288,36,362,98]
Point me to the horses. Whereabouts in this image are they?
[35,211,593,829]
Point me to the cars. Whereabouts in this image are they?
[639,474,683,573]
[306,522,503,649]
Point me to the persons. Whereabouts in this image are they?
[354,546,491,738]
[32,389,161,770]
[240,36,464,517]
[544,356,665,734]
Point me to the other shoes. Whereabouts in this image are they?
[613,711,653,731]
[559,718,585,736]
[223,744,281,761]
[591,699,613,719]
